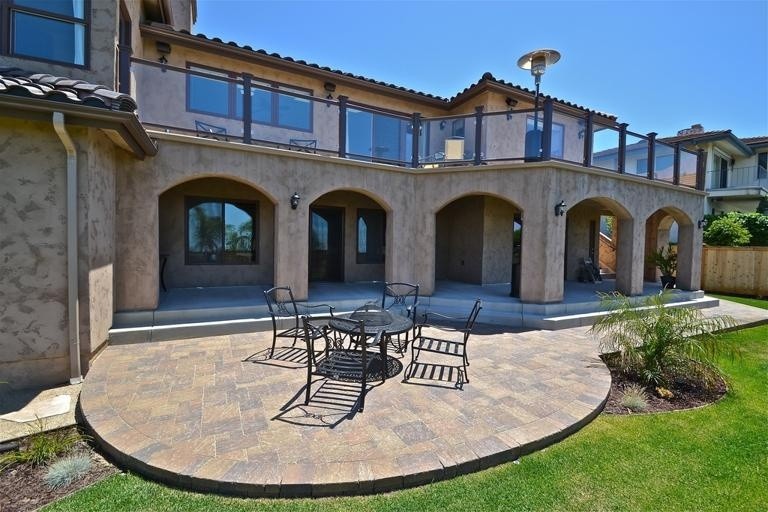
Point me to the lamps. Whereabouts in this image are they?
[410,297,482,384]
[263,285,336,359]
[301,313,385,412]
[382,281,419,352]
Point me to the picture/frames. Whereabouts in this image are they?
[328,311,413,377]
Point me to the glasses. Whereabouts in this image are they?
[291,192,300,209]
[517,48,561,162]
[441,120,447,127]
[730,158,735,165]
[158,55,168,64]
[578,129,585,139]
[505,106,513,120]
[698,217,708,228]
[326,93,333,107]
[555,200,566,216]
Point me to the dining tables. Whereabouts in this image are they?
[646,244,679,289]
